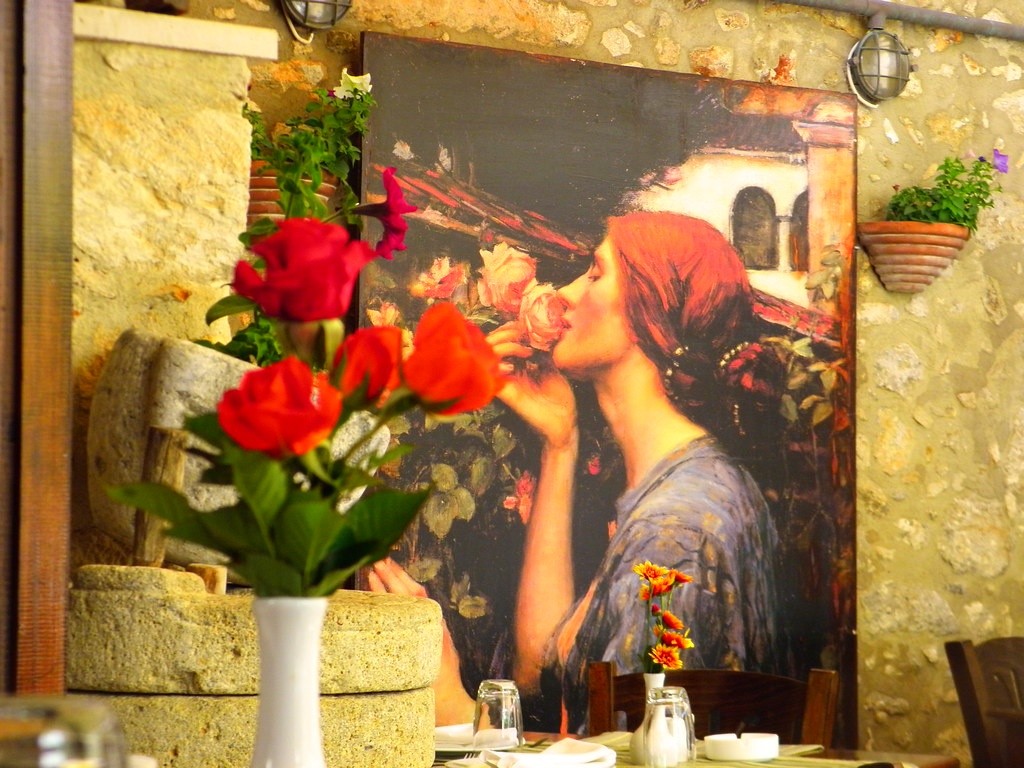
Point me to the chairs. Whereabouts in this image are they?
[944,635,1024,768]
[588,659,839,748]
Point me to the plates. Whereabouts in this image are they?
[704,733,780,763]
[444,757,501,768]
[433,723,529,756]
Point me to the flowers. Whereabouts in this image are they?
[742,242,854,657]
[393,137,534,230]
[367,241,600,688]
[101,171,507,600]
[241,67,376,181]
[631,559,695,674]
[883,149,1008,235]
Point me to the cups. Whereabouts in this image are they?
[472,679,525,749]
[643,685,696,768]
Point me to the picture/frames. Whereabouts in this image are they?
[357,29,860,751]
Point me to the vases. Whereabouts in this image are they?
[629,673,678,767]
[856,221,972,292]
[244,596,330,767]
[246,168,339,238]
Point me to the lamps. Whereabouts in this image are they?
[280,0,352,43]
[845,30,919,109]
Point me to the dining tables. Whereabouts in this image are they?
[430,730,962,768]
